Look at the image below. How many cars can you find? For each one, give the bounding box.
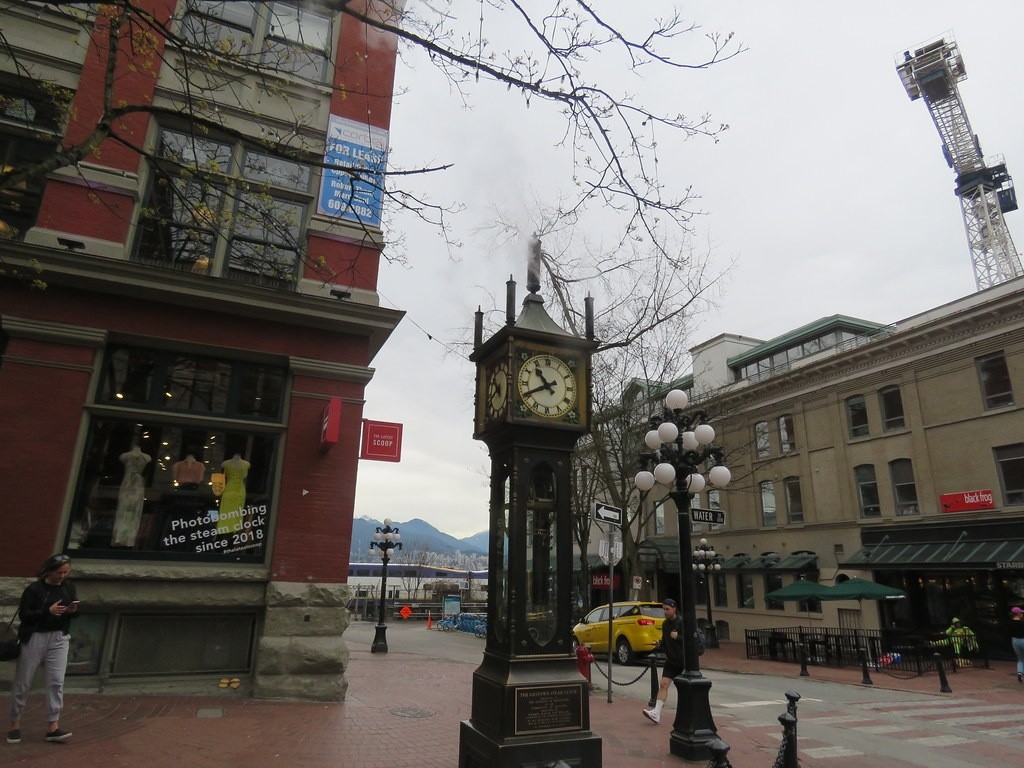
[571,601,681,665]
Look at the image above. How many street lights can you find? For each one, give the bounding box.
[632,388,732,760]
[367,519,402,656]
[691,536,722,649]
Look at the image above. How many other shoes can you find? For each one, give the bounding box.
[1017,672,1023,682]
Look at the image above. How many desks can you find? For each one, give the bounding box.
[796,638,824,663]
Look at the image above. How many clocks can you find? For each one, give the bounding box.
[513,340,586,427]
[485,351,508,427]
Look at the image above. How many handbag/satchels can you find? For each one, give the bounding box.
[0,621,20,662]
[671,616,705,656]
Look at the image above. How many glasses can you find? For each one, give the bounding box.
[49,554,70,562]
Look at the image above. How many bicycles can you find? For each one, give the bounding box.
[437,612,486,639]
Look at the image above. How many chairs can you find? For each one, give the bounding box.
[747,636,769,659]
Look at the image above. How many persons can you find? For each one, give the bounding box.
[172,455,205,483]
[930,618,978,658]
[7,553,80,743]
[110,447,152,547]
[217,454,250,534]
[1010,607,1024,682]
[642,598,682,724]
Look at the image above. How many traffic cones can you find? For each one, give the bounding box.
[427,610,431,629]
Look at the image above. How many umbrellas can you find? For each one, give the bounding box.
[765,578,906,628]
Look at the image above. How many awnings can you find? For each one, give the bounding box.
[839,539,1024,570]
[637,538,679,573]
[526,553,605,571]
[708,555,818,574]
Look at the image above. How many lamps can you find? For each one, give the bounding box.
[864,551,871,562]
[57,237,86,252]
[330,290,352,300]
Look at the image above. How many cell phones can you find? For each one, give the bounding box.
[67,601,80,608]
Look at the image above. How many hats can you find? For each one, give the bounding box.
[663,599,676,607]
[1012,607,1024,613]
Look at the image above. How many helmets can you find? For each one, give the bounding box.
[953,618,960,623]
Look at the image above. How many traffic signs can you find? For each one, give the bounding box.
[593,504,623,525]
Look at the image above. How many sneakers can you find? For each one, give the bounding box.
[45,728,72,741]
[642,709,660,723]
[6,729,22,744]
